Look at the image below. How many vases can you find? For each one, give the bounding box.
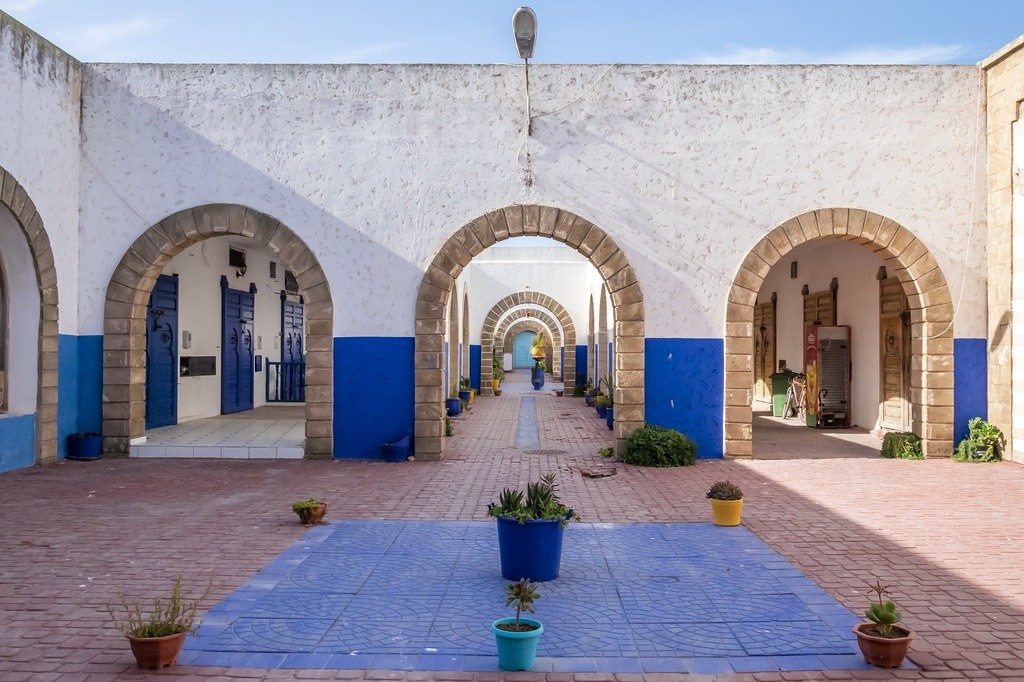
[493,390,502,396]
[382,434,411,463]
[65,429,104,460]
[556,390,564,396]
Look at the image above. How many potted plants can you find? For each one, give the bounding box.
[460,378,477,402]
[621,427,701,467]
[103,564,221,670]
[850,577,916,667]
[487,469,582,581]
[290,497,328,524]
[491,576,546,672]
[529,329,549,390]
[583,366,614,431]
[446,384,462,416]
[878,430,927,460]
[949,416,1005,464]
[705,478,747,527]
[493,354,505,390]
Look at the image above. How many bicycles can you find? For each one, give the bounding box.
[779,367,807,422]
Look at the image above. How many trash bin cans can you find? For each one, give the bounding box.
[65,432,103,459]
[769,371,800,418]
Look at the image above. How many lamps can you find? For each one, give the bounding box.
[236,261,248,279]
[511,5,544,137]
